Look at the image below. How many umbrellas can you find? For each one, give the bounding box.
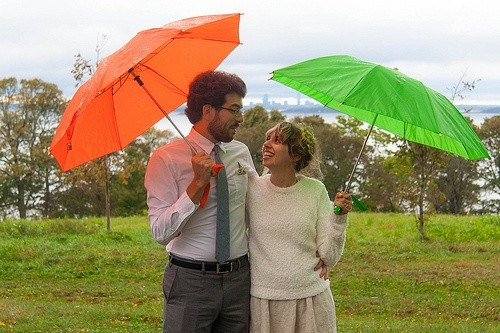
[48,12,244,210]
[267,55,491,215]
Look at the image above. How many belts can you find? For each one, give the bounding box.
[168,254,248,273]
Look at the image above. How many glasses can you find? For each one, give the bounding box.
[213,106,241,116]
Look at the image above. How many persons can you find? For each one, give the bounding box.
[245,122,352,333]
[143,70,338,333]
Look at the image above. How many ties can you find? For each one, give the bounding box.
[213,144,231,265]
